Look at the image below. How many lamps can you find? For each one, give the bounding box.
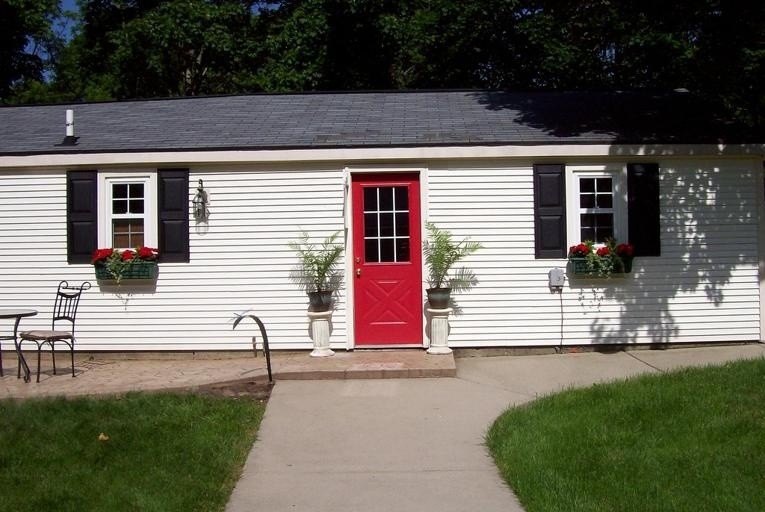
[190,179,210,222]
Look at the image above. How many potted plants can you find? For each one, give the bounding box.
[285,226,348,312]
[419,219,483,310]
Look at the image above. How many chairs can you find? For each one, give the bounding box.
[17,280,92,384]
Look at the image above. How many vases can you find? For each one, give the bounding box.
[95,259,157,282]
[569,256,632,277]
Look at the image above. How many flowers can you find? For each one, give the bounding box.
[90,247,158,287]
[568,239,633,282]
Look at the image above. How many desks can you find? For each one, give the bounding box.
[0,307,38,383]
